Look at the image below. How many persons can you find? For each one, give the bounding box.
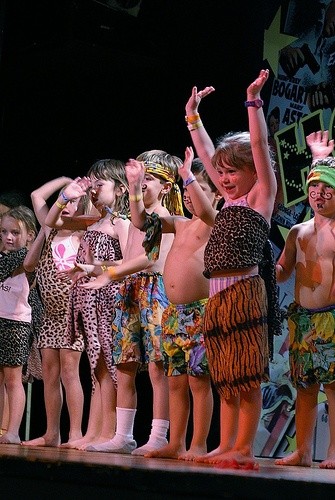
[20,175,86,448]
[275,157,335,470]
[0,205,46,444]
[45,159,131,451]
[267,106,283,153]
[57,150,182,456]
[124,147,223,461]
[185,69,277,465]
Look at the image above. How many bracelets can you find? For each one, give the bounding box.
[101,265,107,273]
[187,121,203,131]
[61,192,71,202]
[185,113,200,123]
[109,268,120,281]
[128,194,143,201]
[183,175,197,187]
[55,201,66,209]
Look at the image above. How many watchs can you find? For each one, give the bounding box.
[244,99,264,108]
